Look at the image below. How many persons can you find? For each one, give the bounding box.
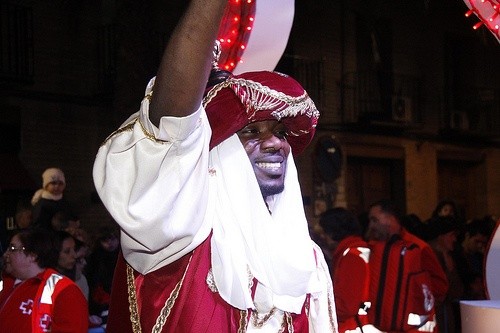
[402,202,500,290]
[0,206,37,249]
[66,215,89,247]
[0,226,88,333]
[92,226,118,328]
[32,168,71,252]
[319,206,372,333]
[368,201,449,333]
[47,232,105,332]
[91,0,337,333]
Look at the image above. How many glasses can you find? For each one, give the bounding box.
[7,244,26,253]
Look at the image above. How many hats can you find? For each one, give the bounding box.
[197,39,320,158]
[42,167,65,189]
